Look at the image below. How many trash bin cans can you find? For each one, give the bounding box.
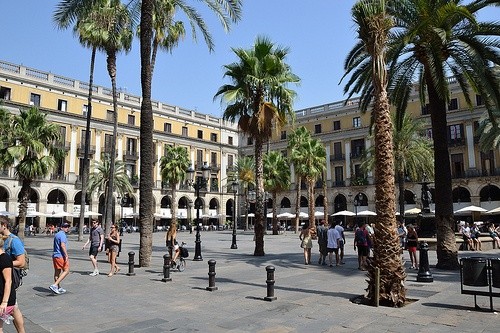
[461,256,489,287]
[491,257,500,289]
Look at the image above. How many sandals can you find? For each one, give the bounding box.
[114,267,121,275]
[108,273,113,277]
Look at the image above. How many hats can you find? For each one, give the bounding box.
[110,226,115,228]
[321,220,326,224]
[61,223,71,227]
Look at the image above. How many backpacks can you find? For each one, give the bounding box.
[9,235,30,278]
[4,253,23,290]
[355,229,366,244]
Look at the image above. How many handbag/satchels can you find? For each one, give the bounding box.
[300,242,304,248]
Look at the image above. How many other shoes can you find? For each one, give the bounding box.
[319,260,327,265]
[173,260,176,264]
[89,271,99,277]
[470,247,475,251]
[476,248,482,251]
[329,263,339,267]
[340,261,346,264]
[358,267,366,271]
[410,266,419,270]
[305,261,311,265]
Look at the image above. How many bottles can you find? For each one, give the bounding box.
[0,312,15,324]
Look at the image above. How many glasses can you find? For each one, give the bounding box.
[92,222,96,224]
[63,225,70,228]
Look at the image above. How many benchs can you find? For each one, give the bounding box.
[456,237,495,243]
[418,238,437,242]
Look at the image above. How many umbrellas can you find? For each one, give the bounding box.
[27,208,106,219]
[197,205,500,219]
[126,213,162,226]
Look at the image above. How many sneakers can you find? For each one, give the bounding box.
[49,284,67,295]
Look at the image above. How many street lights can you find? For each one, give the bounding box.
[230,178,239,249]
[347,191,363,230]
[185,161,213,262]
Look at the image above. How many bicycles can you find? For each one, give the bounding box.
[169,242,186,272]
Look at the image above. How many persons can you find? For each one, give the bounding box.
[48,222,71,295]
[166,222,180,265]
[0,234,25,333]
[0,214,25,268]
[106,222,121,277]
[461,221,500,251]
[81,218,104,276]
[28,223,88,236]
[298,219,421,271]
